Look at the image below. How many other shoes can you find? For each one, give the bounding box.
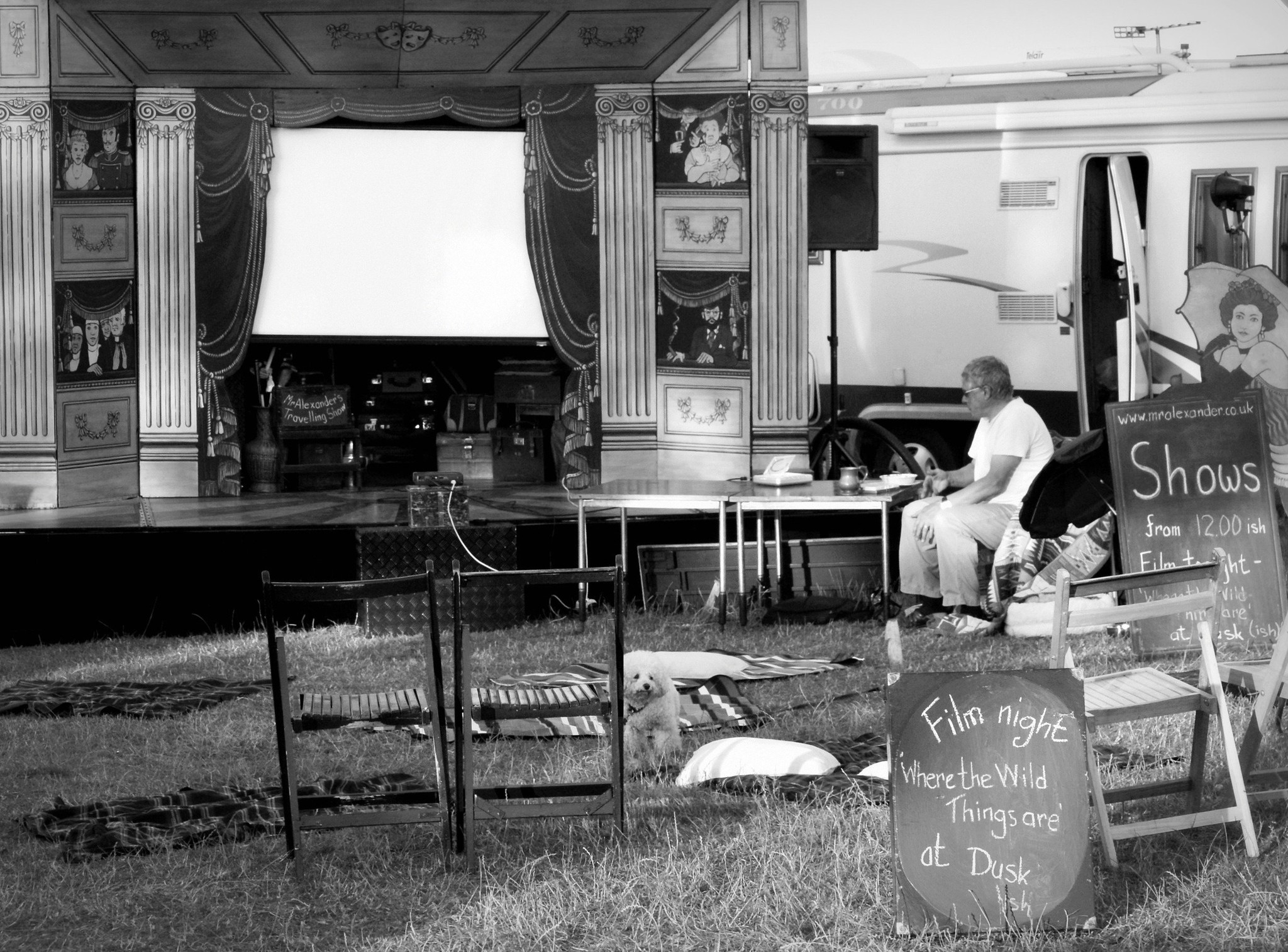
[885,607,932,628]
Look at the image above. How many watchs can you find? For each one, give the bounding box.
[940,497,951,510]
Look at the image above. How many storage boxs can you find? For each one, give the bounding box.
[273,384,351,431]
[436,360,561,481]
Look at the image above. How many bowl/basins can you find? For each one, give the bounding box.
[879,473,918,485]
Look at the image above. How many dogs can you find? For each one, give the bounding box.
[606,651,683,776]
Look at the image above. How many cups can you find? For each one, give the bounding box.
[904,392,912,406]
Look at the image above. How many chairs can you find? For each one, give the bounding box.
[1052,548,1262,869]
[452,555,628,874]
[258,558,452,870]
[1212,610,1288,831]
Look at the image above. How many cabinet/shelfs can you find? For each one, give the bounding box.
[278,422,364,493]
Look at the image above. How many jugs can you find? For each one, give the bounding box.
[838,465,869,496]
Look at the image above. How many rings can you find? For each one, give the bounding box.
[924,525,928,529]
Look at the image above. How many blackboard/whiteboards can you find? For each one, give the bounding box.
[1104,388,1286,656]
[880,665,1101,936]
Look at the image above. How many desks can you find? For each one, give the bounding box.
[570,478,924,633]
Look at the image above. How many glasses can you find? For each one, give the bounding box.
[963,387,982,398]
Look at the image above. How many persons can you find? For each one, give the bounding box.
[894,356,1051,631]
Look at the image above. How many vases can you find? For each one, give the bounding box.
[247,405,281,492]
